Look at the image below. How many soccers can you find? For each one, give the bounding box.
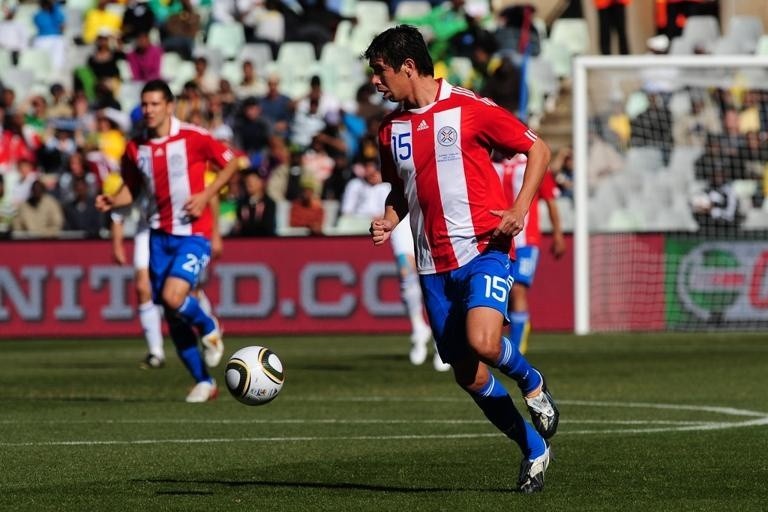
[225,346,284,405]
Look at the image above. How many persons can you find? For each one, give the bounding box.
[362,23,560,494]
[386,214,452,372]
[118,103,225,371]
[93,76,240,403]
[489,118,567,357]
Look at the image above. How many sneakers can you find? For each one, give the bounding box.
[184,377,217,404]
[139,353,166,370]
[197,289,211,316]
[432,345,451,372]
[411,328,430,366]
[200,316,224,368]
[521,366,559,438]
[517,437,553,492]
